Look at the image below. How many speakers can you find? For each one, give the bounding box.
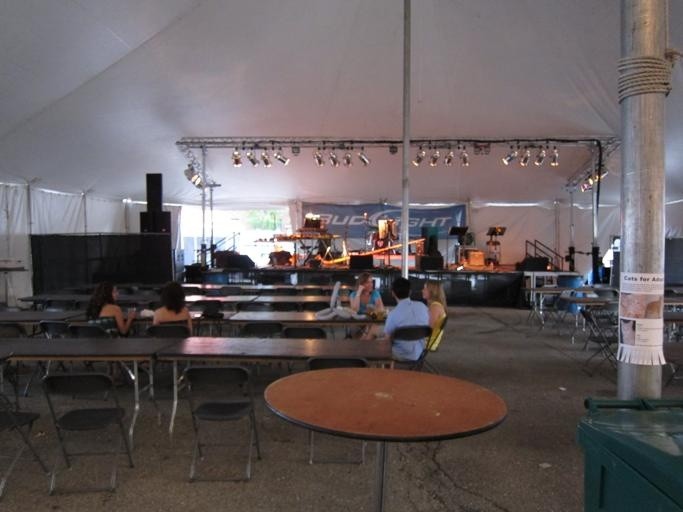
[139,211,154,233]
[146,173,163,212]
[155,211,171,233]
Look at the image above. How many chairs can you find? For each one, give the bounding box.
[191,300,221,310]
[270,302,301,332]
[279,327,327,373]
[578,308,618,377]
[182,286,198,294]
[217,286,243,296]
[420,314,450,374]
[273,287,298,295]
[40,369,134,493]
[159,325,192,425]
[302,300,329,312]
[304,356,369,369]
[301,287,323,295]
[0,359,52,499]
[374,324,433,369]
[182,366,262,481]
[238,322,282,336]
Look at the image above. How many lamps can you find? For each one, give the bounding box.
[550,148,559,166]
[445,151,455,166]
[580,167,608,193]
[185,168,197,182]
[312,151,325,168]
[411,150,426,167]
[520,150,530,167]
[356,152,370,166]
[260,152,272,167]
[460,151,471,168]
[194,176,201,187]
[273,152,290,166]
[231,151,242,168]
[328,151,339,168]
[246,153,259,167]
[342,153,351,166]
[501,150,517,167]
[430,151,440,168]
[533,150,547,166]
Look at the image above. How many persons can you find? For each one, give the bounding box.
[85,281,137,338]
[421,281,449,351]
[152,282,193,337]
[350,273,385,339]
[366,277,430,363]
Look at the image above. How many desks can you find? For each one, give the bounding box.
[260,284,350,295]
[121,308,236,322]
[0,337,175,464]
[185,294,255,306]
[155,335,394,447]
[0,261,29,310]
[554,297,611,330]
[228,309,385,324]
[67,283,99,292]
[526,288,589,326]
[17,292,95,310]
[180,283,260,294]
[254,294,332,305]
[264,366,508,511]
[2,305,87,338]
